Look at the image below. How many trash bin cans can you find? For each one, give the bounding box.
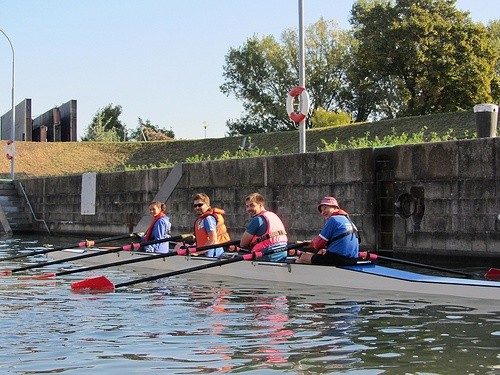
[473,103,499,137]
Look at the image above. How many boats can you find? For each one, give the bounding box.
[46,246,500,308]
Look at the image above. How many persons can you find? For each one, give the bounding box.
[133,201,172,254]
[68,273,360,375]
[239,192,288,262]
[174,193,231,258]
[295,196,360,266]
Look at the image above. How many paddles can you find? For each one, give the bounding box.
[69,240,308,295]
[16,239,236,281]
[358,251,500,282]
[0,233,193,277]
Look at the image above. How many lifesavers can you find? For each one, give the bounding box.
[5,140,15,160]
[285,86,310,123]
